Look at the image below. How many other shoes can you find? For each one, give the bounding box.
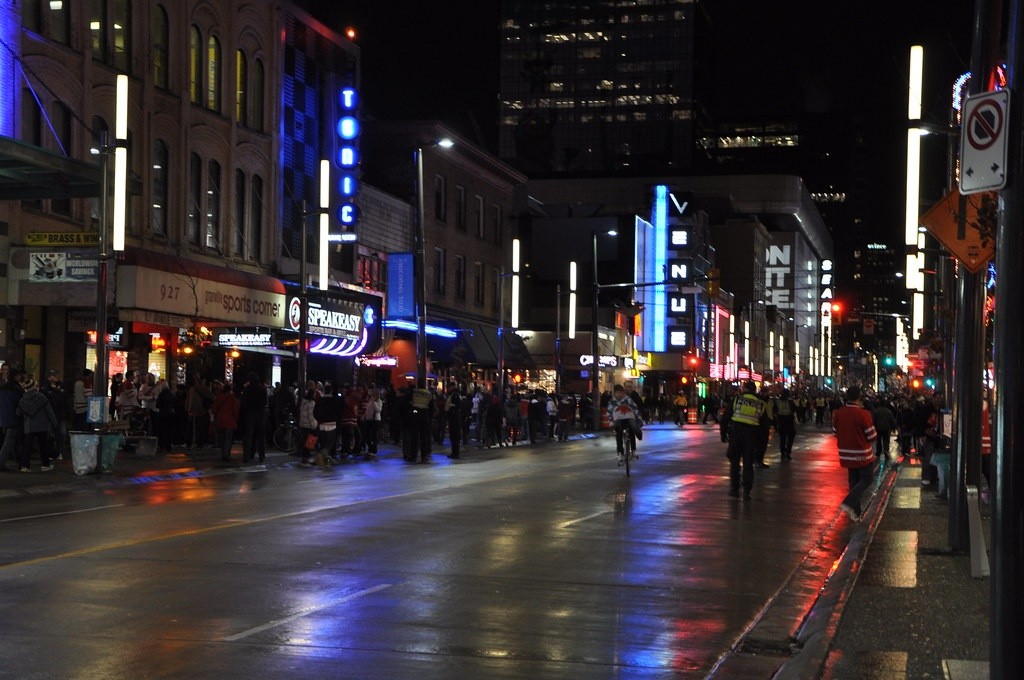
[780,447,790,456]
[21,466,32,473]
[840,503,859,522]
[632,450,635,456]
[289,443,379,468]
[730,487,739,497]
[433,436,570,458]
[755,463,769,469]
[743,488,750,497]
[41,465,55,472]
[618,452,625,462]
[637,433,642,440]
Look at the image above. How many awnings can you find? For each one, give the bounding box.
[451,318,537,368]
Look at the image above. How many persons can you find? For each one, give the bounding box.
[608,384,642,467]
[597,383,947,469]
[834,386,876,522]
[0,361,266,473]
[721,382,766,503]
[270,379,594,468]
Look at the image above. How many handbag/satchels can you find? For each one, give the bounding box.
[303,433,318,450]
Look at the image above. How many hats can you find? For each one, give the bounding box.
[134,371,142,377]
[25,379,38,392]
[48,369,56,376]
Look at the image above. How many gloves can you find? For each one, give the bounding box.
[609,422,614,428]
[720,433,728,442]
[638,416,642,427]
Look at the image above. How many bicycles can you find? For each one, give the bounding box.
[613,423,639,478]
[272,407,302,453]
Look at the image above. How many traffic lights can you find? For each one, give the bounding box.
[690,358,696,364]
[831,304,841,311]
[925,379,934,387]
[912,380,922,388]
[886,358,892,365]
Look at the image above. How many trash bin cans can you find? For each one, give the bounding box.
[934,448,950,498]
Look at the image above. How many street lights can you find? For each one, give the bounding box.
[590,228,618,432]
[413,139,455,462]
[87,145,110,478]
[894,44,972,554]
[556,261,578,392]
[496,239,519,446]
